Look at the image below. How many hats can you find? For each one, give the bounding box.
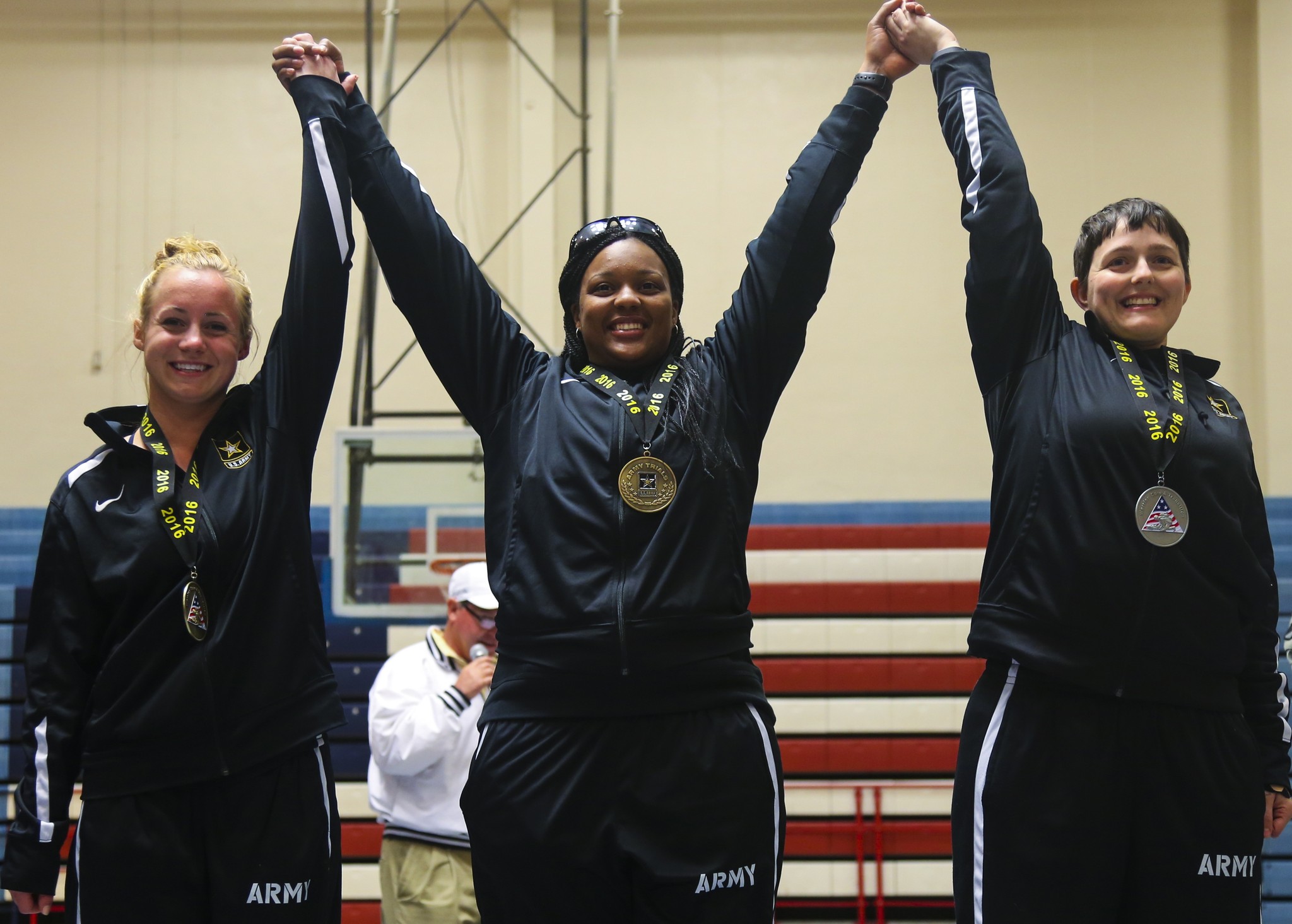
[449,561,499,610]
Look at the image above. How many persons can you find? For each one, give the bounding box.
[367,561,499,924]
[0,32,358,924]
[274,0,928,924]
[885,0,1292,924]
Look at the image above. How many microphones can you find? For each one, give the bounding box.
[470,643,488,660]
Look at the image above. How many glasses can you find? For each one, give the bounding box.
[463,601,497,630]
[569,216,669,258]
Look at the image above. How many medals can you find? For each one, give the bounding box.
[618,456,677,512]
[183,581,209,641]
[1135,486,1189,547]
